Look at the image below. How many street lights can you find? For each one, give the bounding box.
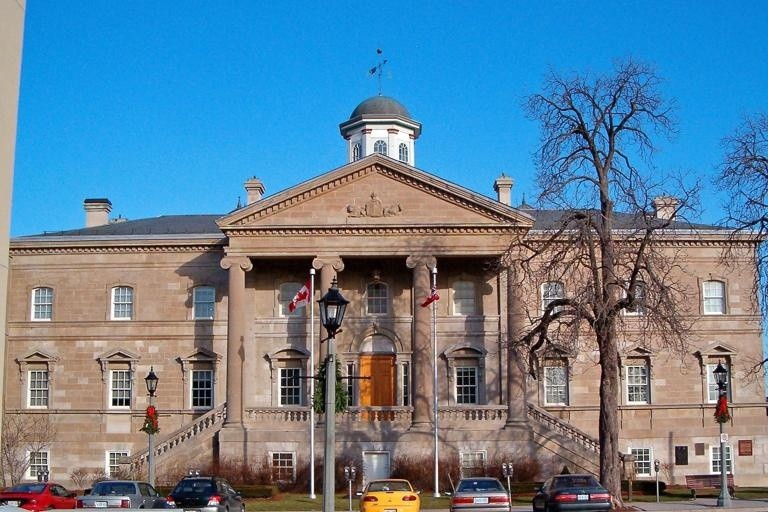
[712,358,733,507]
[144,365,159,488]
[315,273,351,511]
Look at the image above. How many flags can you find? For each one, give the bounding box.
[419,281,441,307]
[288,276,316,314]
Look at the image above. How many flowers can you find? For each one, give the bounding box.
[139,405,160,434]
[713,395,730,423]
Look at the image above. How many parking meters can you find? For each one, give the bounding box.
[344,466,356,511]
[502,462,514,511]
[37,469,50,483]
[654,460,660,500]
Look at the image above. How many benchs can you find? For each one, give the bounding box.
[686,475,735,500]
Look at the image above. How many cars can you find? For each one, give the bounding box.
[74,480,167,509]
[355,479,421,512]
[1,482,84,512]
[532,473,612,511]
[165,476,246,512]
[444,475,510,512]
